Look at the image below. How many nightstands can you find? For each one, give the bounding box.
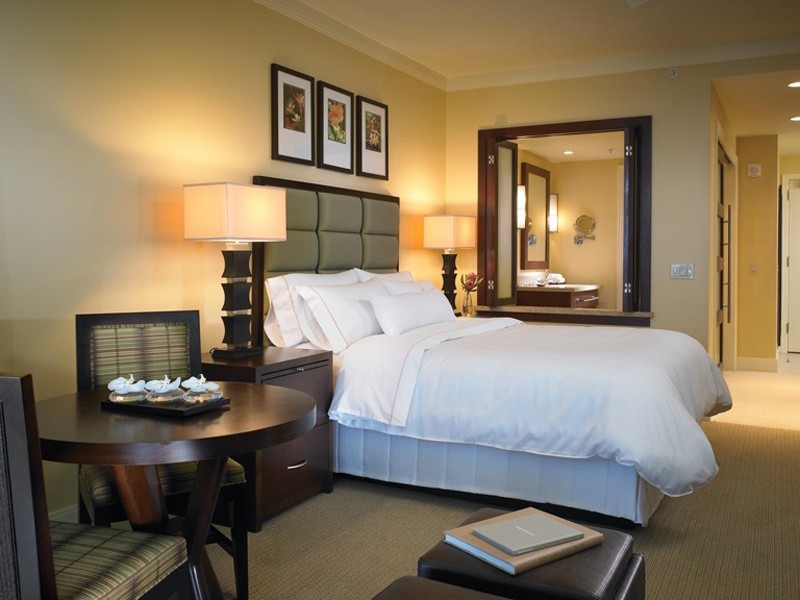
[198,346,334,532]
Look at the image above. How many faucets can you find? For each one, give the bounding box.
[536,278,559,286]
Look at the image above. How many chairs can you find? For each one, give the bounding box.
[0,373,188,600]
[75,310,248,600]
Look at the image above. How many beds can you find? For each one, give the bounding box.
[251,176,733,535]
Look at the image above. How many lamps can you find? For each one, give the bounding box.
[183,182,287,360]
[423,216,478,310]
[573,214,596,244]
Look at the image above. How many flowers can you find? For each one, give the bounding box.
[459,271,484,314]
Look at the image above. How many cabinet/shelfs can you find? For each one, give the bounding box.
[515,284,600,308]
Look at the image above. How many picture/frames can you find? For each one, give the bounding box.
[355,95,389,180]
[317,80,354,174]
[269,63,316,166]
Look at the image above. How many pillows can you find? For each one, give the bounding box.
[264,266,457,354]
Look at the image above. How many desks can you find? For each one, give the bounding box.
[31,381,317,600]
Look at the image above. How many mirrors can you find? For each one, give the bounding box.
[521,162,550,270]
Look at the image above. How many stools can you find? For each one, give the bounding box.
[371,507,646,600]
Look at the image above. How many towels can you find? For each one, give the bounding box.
[547,273,566,284]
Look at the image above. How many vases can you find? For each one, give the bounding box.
[462,293,475,317]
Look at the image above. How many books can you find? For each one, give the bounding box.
[442,506,604,576]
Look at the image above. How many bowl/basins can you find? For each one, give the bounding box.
[183,388,223,403]
[109,388,148,402]
[146,387,185,403]
